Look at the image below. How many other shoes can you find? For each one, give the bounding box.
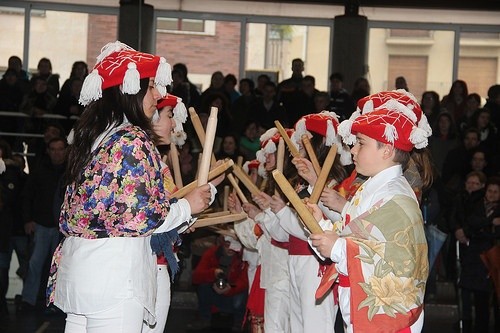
[18,303,33,313]
[187,319,210,329]
[232,317,241,332]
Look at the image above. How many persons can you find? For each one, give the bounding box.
[46,40,212,333]
[0,56,89,333]
[142,58,371,333]
[297,99,429,333]
[395,75,500,333]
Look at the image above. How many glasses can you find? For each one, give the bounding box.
[423,97,433,101]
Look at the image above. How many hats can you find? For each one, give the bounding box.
[78,41,174,107]
[242,160,260,175]
[291,110,353,166]
[149,93,188,132]
[256,128,294,162]
[336,89,433,151]
